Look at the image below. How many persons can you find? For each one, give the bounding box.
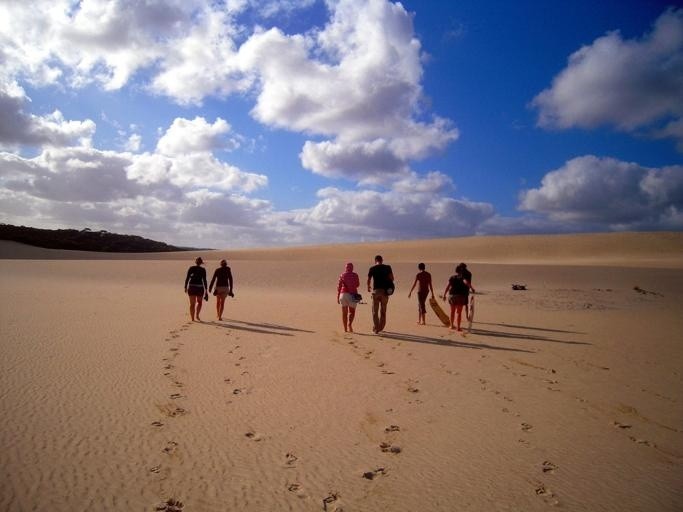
[408,263,433,325]
[337,263,360,331]
[185,257,208,321]
[443,266,475,331]
[209,260,234,320]
[367,255,394,334]
[460,263,471,320]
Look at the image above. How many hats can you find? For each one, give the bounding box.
[195,257,204,264]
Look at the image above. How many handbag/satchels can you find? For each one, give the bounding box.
[340,275,362,303]
